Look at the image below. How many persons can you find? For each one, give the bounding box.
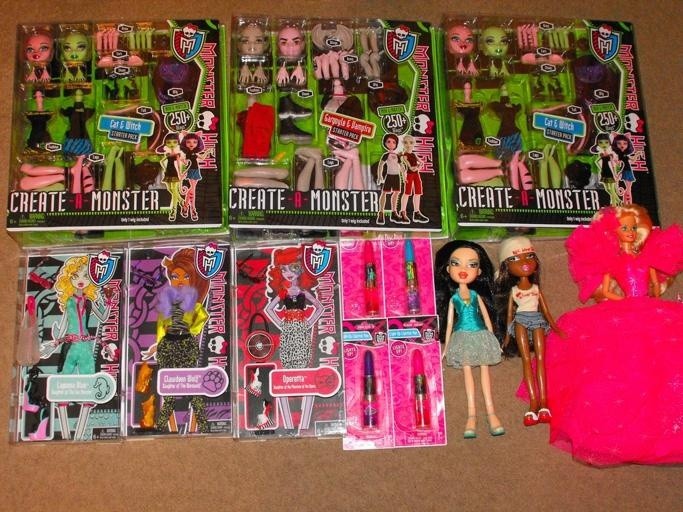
[492,236,569,425]
[232,27,383,190]
[434,242,512,438]
[515,203,683,467]
[448,24,572,190]
[19,25,154,191]
[9,16,683,472]
